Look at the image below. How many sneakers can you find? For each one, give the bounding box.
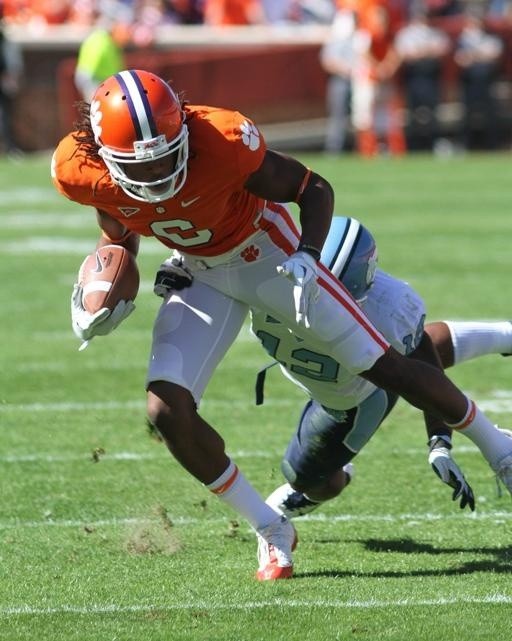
[263,464,356,519]
[486,425,512,499]
[255,519,299,582]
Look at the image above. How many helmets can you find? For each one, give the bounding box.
[319,215,377,302]
[90,70,188,207]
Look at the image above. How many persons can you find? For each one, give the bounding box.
[260,214,511,522]
[1,0,511,154]
[49,68,511,588]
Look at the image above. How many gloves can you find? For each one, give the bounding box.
[71,284,136,341]
[428,451,477,513]
[153,257,191,296]
[277,253,321,330]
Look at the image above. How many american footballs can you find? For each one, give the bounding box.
[77,245,138,314]
